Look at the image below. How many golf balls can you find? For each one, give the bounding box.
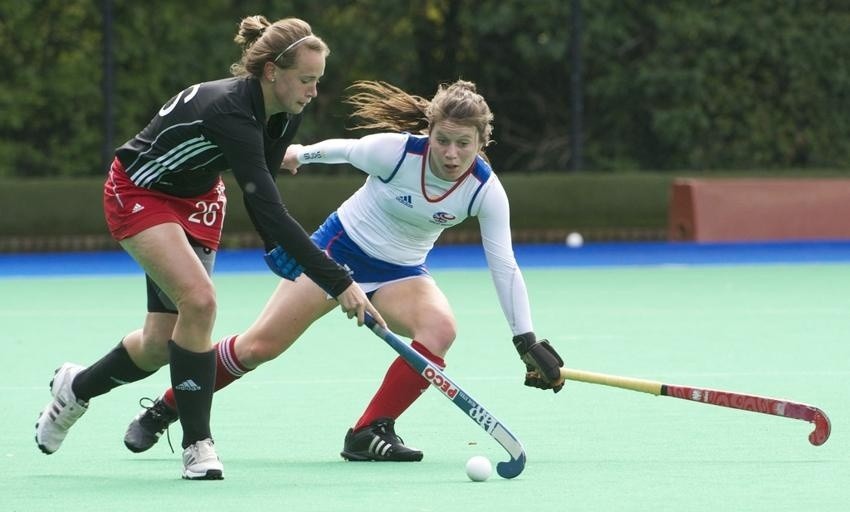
[566,233,583,249]
[464,456,491,482]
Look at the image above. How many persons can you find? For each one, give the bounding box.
[33,14,387,480]
[124,81,565,462]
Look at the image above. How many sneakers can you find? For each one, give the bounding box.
[123,396,179,454]
[33,362,89,456]
[340,418,423,462]
[180,437,224,480]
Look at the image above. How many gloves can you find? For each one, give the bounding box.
[264,245,306,282]
[512,331,566,394]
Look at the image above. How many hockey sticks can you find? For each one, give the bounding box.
[354,310,526,478]
[528,365,831,446]
